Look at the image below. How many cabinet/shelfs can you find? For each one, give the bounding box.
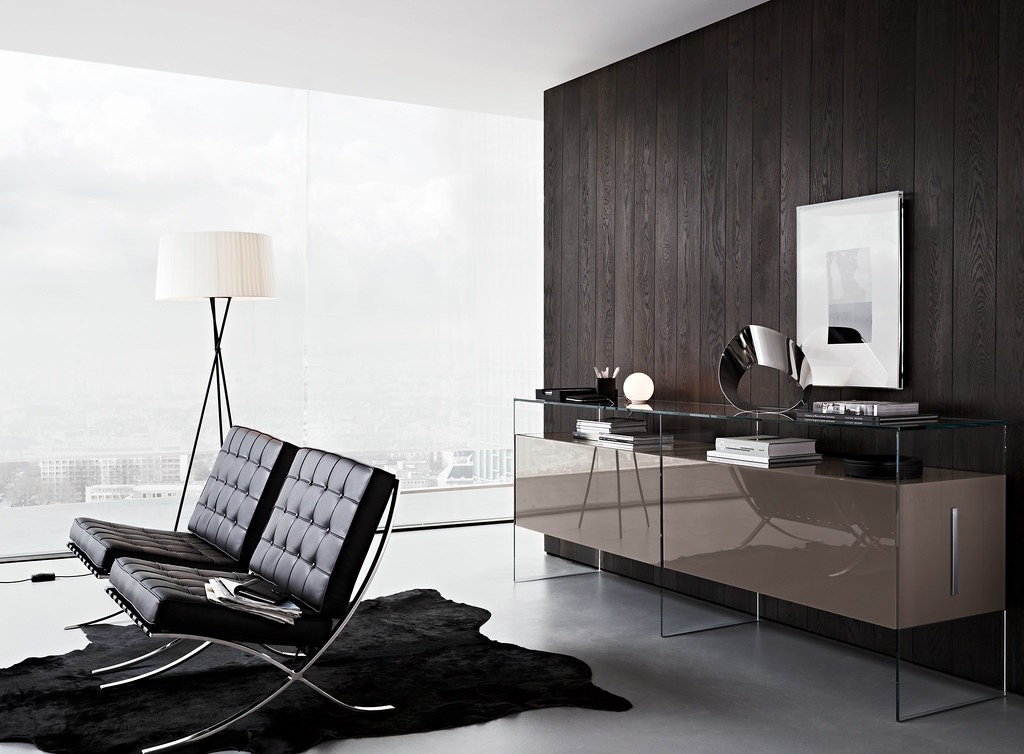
[513,397,1008,726]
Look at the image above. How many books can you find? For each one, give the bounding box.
[203,576,303,626]
[565,395,609,404]
[572,418,674,452]
[796,399,938,424]
[707,434,823,470]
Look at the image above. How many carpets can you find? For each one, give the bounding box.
[1,588,636,753]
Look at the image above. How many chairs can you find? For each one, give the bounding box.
[99,447,397,754]
[67,425,301,676]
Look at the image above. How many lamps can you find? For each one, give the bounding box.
[152,229,276,534]
